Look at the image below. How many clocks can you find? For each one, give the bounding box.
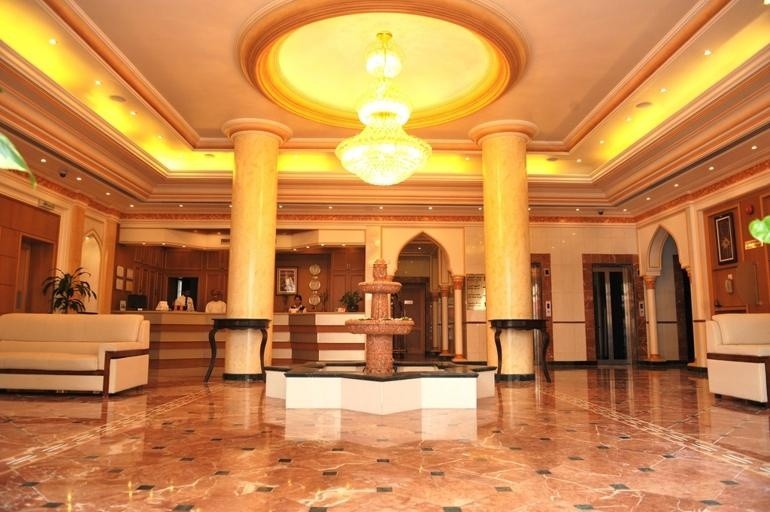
[308,264,322,306]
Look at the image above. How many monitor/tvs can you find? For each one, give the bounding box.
[128,294,147,310]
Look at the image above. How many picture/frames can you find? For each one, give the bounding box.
[276,266,299,296]
[714,211,737,266]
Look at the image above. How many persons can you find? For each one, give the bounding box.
[174,287,194,311]
[205,288,227,313]
[288,294,308,313]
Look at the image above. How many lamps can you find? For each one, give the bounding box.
[333,32,433,187]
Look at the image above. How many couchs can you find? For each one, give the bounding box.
[704,312,769,407]
[0,313,150,396]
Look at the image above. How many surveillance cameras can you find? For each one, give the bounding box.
[597,209,604,215]
[59,170,68,177]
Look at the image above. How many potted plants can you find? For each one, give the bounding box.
[339,289,362,311]
